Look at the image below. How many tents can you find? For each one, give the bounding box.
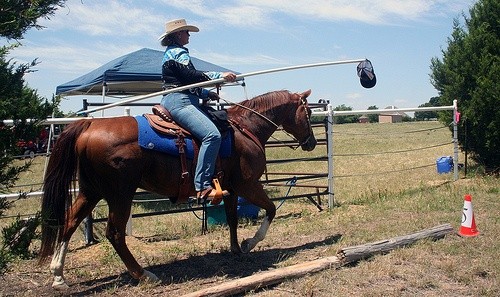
[40,47,250,193]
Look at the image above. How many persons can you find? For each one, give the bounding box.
[159,18,237,204]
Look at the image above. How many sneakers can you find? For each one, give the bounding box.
[197,187,230,204]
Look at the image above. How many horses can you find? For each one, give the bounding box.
[35,89,317,290]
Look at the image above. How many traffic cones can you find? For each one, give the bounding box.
[459,194,481,237]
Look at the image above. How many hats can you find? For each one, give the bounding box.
[159,19,199,40]
[357,59,376,88]
